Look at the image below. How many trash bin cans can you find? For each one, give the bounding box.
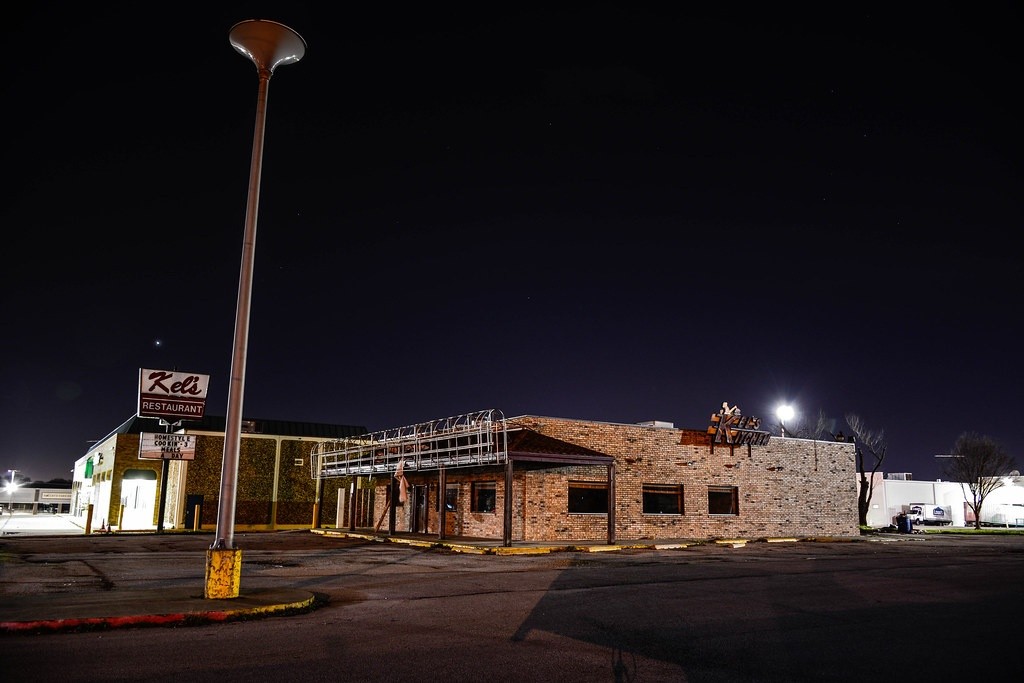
[897,517,910,534]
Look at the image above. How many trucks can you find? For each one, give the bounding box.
[905,503,953,525]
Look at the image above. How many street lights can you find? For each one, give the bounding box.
[206,18,307,599]
[7,469,21,515]
[776,406,794,437]
